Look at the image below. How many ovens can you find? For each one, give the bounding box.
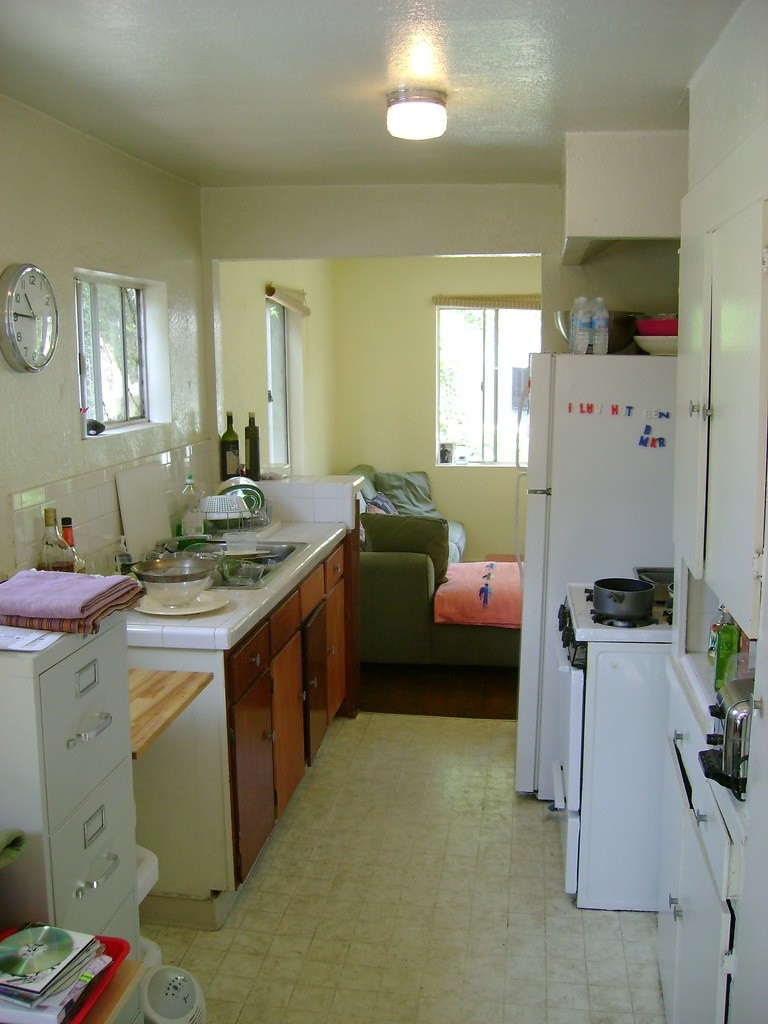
[551,642,586,893]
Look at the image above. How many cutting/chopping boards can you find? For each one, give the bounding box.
[127,668,215,760]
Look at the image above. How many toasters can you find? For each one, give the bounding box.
[698,676,754,802]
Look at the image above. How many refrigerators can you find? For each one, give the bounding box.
[512,353,678,804]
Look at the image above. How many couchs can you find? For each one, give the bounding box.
[343,464,520,667]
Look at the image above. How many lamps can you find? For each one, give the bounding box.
[386,86,448,140]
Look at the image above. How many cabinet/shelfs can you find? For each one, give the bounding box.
[0,607,141,962]
[666,121,768,1024]
[127,539,347,932]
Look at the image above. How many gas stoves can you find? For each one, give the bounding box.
[557,582,672,668]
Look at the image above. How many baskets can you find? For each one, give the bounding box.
[203,495,253,520]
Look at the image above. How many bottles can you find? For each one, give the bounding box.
[220,411,240,481]
[174,474,208,537]
[569,296,609,355]
[714,608,739,693]
[244,412,260,481]
[707,605,726,667]
[61,516,86,575]
[36,508,75,573]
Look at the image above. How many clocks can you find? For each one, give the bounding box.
[0,263,59,373]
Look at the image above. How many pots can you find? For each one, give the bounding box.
[592,577,656,620]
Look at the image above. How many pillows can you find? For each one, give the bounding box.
[360,513,449,584]
[371,471,437,516]
[369,491,398,516]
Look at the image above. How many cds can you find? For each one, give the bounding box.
[0,926,74,975]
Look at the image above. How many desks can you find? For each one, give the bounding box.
[128,668,215,760]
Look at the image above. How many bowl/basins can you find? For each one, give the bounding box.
[222,558,265,586]
[635,336,678,356]
[554,310,646,354]
[223,531,257,554]
[182,543,224,569]
[635,320,678,335]
[130,556,219,609]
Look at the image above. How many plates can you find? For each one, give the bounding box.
[217,484,265,519]
[133,590,230,616]
